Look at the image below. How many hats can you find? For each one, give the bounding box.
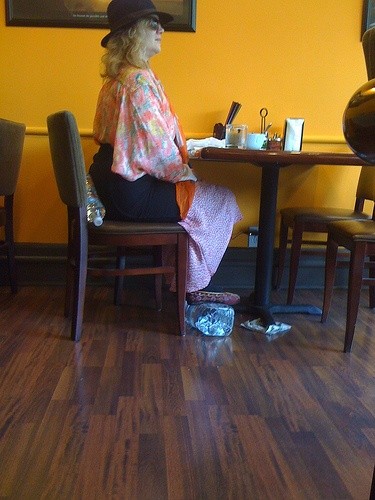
[101,0,174,48]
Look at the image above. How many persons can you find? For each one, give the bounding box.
[88,0,243,295]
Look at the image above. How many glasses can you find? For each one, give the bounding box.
[147,19,160,28]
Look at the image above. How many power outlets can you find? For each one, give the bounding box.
[248,226,258,248]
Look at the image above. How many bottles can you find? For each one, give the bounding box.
[184,303,234,337]
[85,174,106,226]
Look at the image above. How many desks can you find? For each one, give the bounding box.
[185,139,375,328]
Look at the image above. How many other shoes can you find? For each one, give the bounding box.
[186,290,241,305]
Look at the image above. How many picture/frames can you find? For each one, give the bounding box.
[5,0,196,32]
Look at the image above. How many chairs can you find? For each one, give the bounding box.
[47,110,189,342]
[274,165,375,305]
[0,118,26,295]
[321,207,375,353]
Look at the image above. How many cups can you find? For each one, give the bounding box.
[248,133,268,150]
[225,124,247,150]
